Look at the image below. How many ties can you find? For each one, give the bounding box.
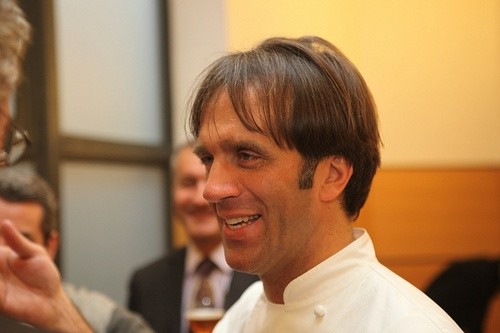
[194,258,215,309]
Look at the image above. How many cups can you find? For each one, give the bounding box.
[186,308,223,333]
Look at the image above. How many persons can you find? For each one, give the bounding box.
[0,35,469,333]
[0,160,155,333]
[127,141,261,333]
[0,0,34,169]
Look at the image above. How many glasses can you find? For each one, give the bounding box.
[0,105,34,166]
